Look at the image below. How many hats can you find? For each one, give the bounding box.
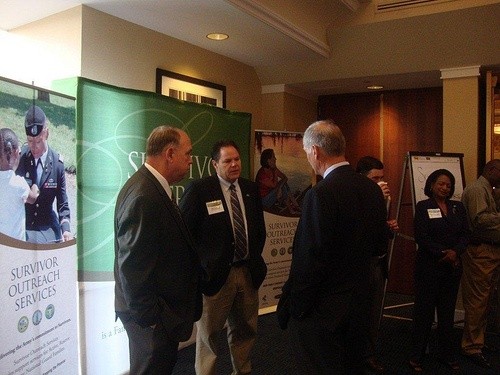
[25,105,46,137]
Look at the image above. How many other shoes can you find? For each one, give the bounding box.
[365,357,384,373]
[471,354,490,368]
[444,358,460,370]
[407,358,423,372]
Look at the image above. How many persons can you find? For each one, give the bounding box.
[0,104,73,244]
[178,140,266,375]
[409,169,469,373]
[114,125,204,375]
[255,149,291,210]
[275,120,399,375]
[461,160,500,371]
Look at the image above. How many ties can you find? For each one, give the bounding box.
[228,185,247,260]
[171,188,184,225]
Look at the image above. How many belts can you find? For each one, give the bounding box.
[481,239,499,246]
[231,260,248,266]
